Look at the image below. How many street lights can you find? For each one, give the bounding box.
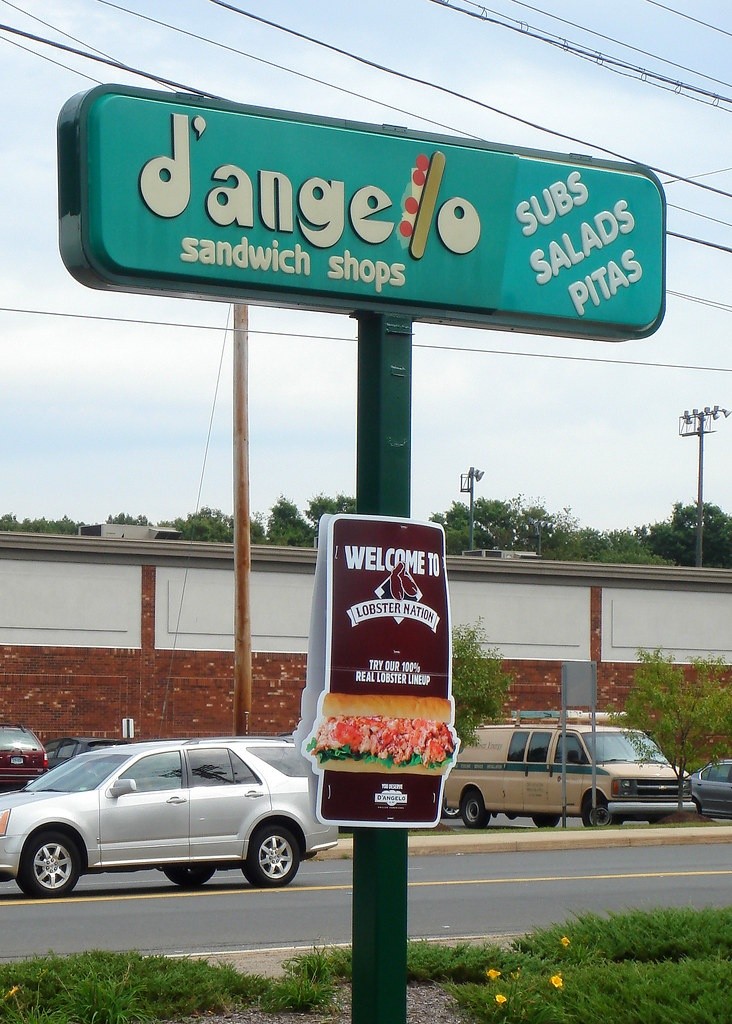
[680,405,732,566]
[461,467,486,550]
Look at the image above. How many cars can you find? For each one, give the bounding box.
[690,757,732,820]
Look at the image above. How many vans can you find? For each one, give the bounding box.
[443,722,699,832]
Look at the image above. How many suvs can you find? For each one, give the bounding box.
[42,734,131,778]
[0,734,340,900]
[0,722,50,790]
